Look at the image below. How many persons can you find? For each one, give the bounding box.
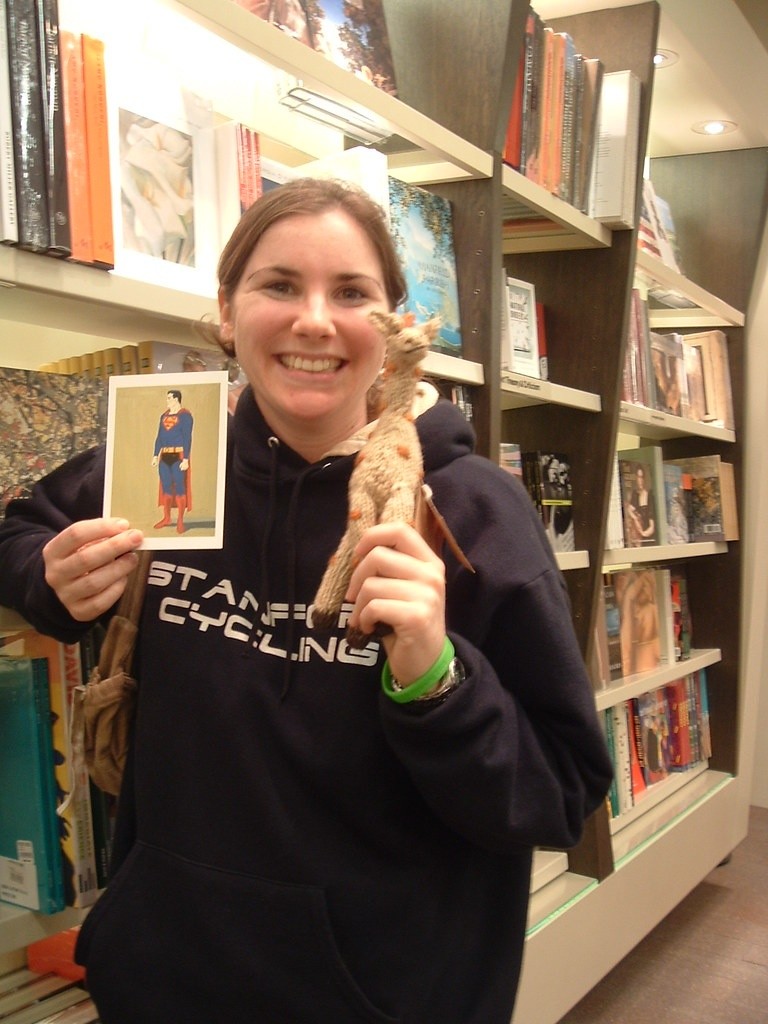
[0,177,613,1024]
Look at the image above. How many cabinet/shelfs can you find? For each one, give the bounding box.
[0,0,768,1024]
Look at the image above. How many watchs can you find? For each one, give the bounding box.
[391,657,465,701]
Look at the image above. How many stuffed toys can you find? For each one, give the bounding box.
[315,311,445,615]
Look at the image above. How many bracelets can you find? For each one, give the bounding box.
[382,634,455,703]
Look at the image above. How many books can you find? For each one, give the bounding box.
[594,566,695,693]
[0,0,465,358]
[0,608,115,921]
[436,267,736,430]
[498,442,739,551]
[0,339,247,497]
[600,668,712,817]
[499,0,687,277]
